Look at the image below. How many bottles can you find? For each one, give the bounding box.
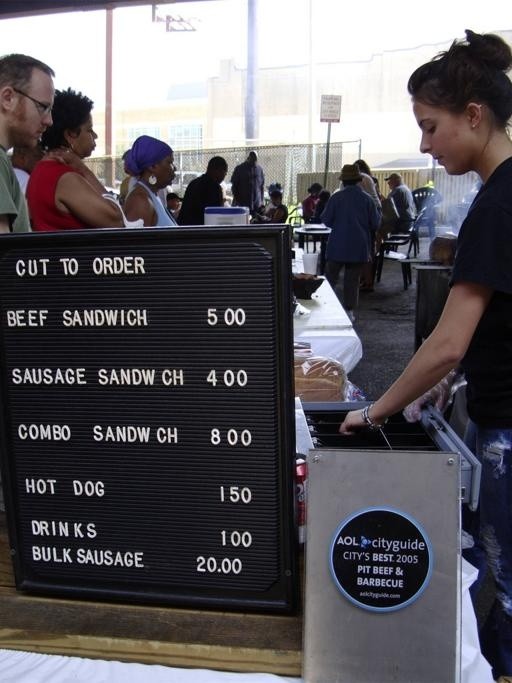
[297,201,303,216]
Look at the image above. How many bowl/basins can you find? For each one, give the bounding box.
[291,273,325,300]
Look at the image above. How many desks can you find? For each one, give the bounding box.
[294,224,340,267]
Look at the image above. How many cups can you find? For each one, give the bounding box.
[303,252,319,275]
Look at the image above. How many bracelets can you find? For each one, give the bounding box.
[363,402,388,431]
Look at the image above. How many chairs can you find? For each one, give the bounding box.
[409,186,444,236]
[373,211,420,289]
[389,205,429,260]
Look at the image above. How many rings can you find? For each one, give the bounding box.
[65,147,69,152]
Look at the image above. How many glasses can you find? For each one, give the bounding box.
[12,86,54,117]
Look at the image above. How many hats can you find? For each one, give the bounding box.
[338,165,364,181]
[384,173,400,180]
[268,183,283,195]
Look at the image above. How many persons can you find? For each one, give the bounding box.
[12,137,47,194]
[25,86,125,233]
[229,148,418,324]
[0,54,58,235]
[119,134,228,226]
[339,30,512,672]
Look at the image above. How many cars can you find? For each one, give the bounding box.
[93,170,271,207]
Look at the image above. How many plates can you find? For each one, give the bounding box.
[304,223,327,229]
